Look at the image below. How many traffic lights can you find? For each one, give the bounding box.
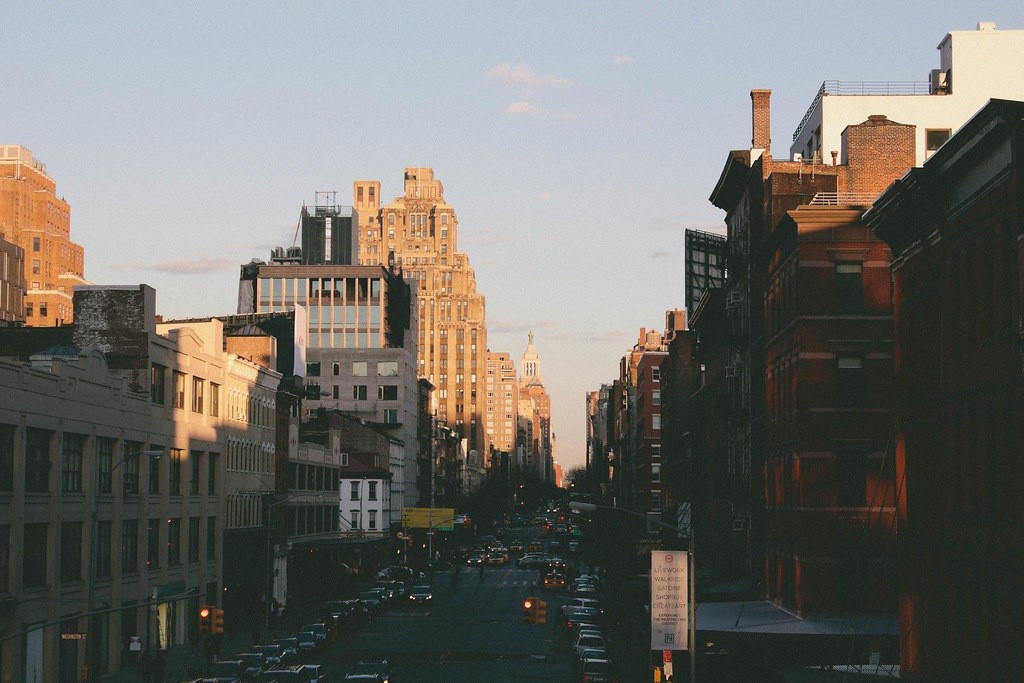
[197,605,211,633]
[520,597,549,626]
[211,606,224,635]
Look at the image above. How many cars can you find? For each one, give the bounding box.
[189,577,432,683]
[460,494,616,683]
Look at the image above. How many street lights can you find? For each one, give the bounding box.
[568,501,695,683]
[264,491,324,641]
[77,448,168,682]
